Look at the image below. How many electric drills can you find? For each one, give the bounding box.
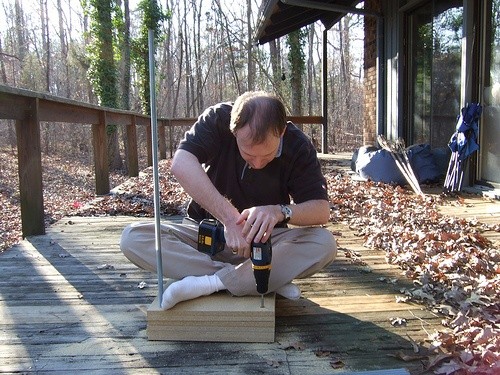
[197,219,272,307]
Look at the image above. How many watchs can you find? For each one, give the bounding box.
[278,204,292,224]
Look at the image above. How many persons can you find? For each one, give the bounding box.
[119,90,338,313]
